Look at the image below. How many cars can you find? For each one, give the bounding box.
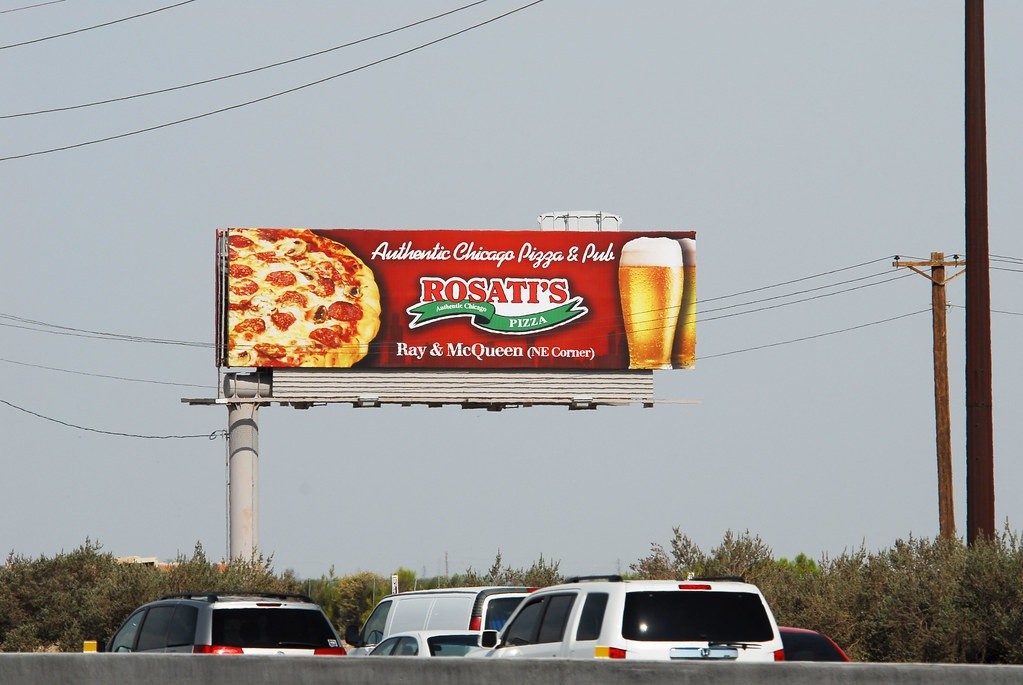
[779,626,851,662]
[367,629,486,658]
[346,586,547,656]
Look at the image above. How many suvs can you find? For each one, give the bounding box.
[478,574,787,663]
[106,591,347,655]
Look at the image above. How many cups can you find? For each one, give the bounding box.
[618,247,696,371]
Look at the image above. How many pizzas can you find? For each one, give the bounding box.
[228,227,381,368]
